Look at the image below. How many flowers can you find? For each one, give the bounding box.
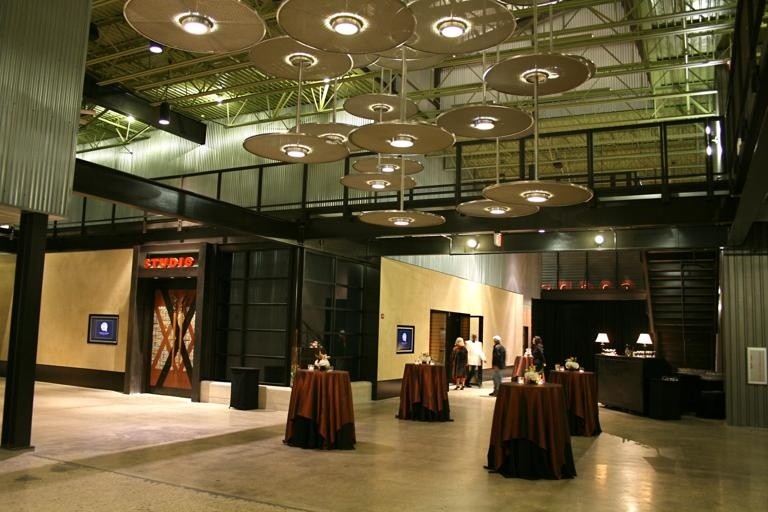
[413,352,432,365]
[522,365,547,385]
[308,340,336,371]
[564,356,580,370]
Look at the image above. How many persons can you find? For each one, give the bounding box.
[488,336,507,398]
[530,336,547,372]
[464,336,488,389]
[451,337,469,390]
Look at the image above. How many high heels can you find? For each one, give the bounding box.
[461,385,466,390]
[455,386,460,391]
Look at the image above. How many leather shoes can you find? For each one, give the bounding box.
[466,382,473,389]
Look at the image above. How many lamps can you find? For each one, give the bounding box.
[276,0,417,55]
[287,78,363,152]
[158,68,171,126]
[352,157,424,175]
[149,41,164,54]
[595,333,609,354]
[123,0,267,54]
[340,67,419,194]
[632,333,655,358]
[242,36,353,164]
[457,44,538,218]
[394,1,517,56]
[544,3,597,81]
[342,94,419,122]
[347,46,456,230]
[436,48,534,142]
[483,1,595,208]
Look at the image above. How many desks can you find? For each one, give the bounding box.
[483,382,577,479]
[550,370,603,437]
[395,362,455,422]
[511,355,534,382]
[281,368,357,450]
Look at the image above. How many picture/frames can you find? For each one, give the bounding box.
[86,314,120,345]
[746,346,768,384]
[396,325,416,354]
